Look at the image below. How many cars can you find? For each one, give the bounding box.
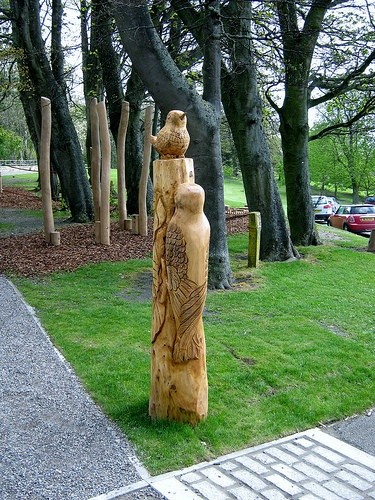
[311,195,375,233]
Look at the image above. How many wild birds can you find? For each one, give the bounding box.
[151,110,191,157]
